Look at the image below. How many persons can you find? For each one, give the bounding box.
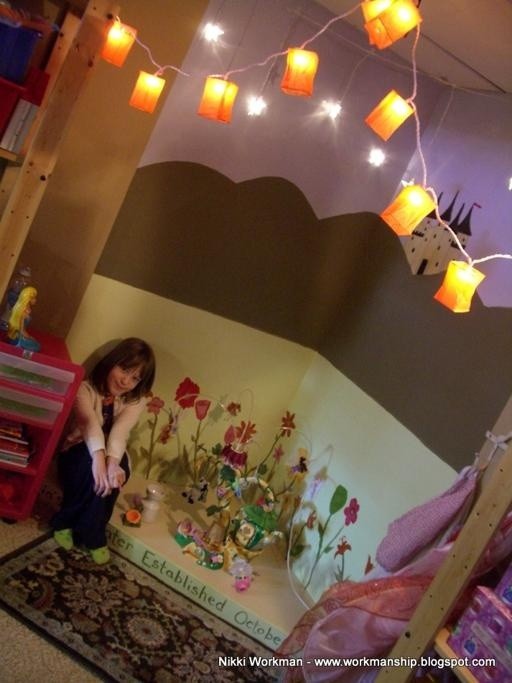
[5,285,44,352]
[50,336,157,567]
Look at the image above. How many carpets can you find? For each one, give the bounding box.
[1,527,287,682]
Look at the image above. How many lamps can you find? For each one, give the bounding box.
[99,1,512,318]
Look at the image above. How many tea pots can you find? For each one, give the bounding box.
[229,500,283,552]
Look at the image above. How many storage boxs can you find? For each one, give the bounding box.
[450,584,511,682]
[0,77,23,143]
[1,13,44,86]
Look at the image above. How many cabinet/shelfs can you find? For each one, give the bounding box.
[0,0,106,311]
[0,321,82,520]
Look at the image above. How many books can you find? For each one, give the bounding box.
[0,417,38,469]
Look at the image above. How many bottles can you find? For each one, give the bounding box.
[0,270,31,331]
[141,483,165,523]
